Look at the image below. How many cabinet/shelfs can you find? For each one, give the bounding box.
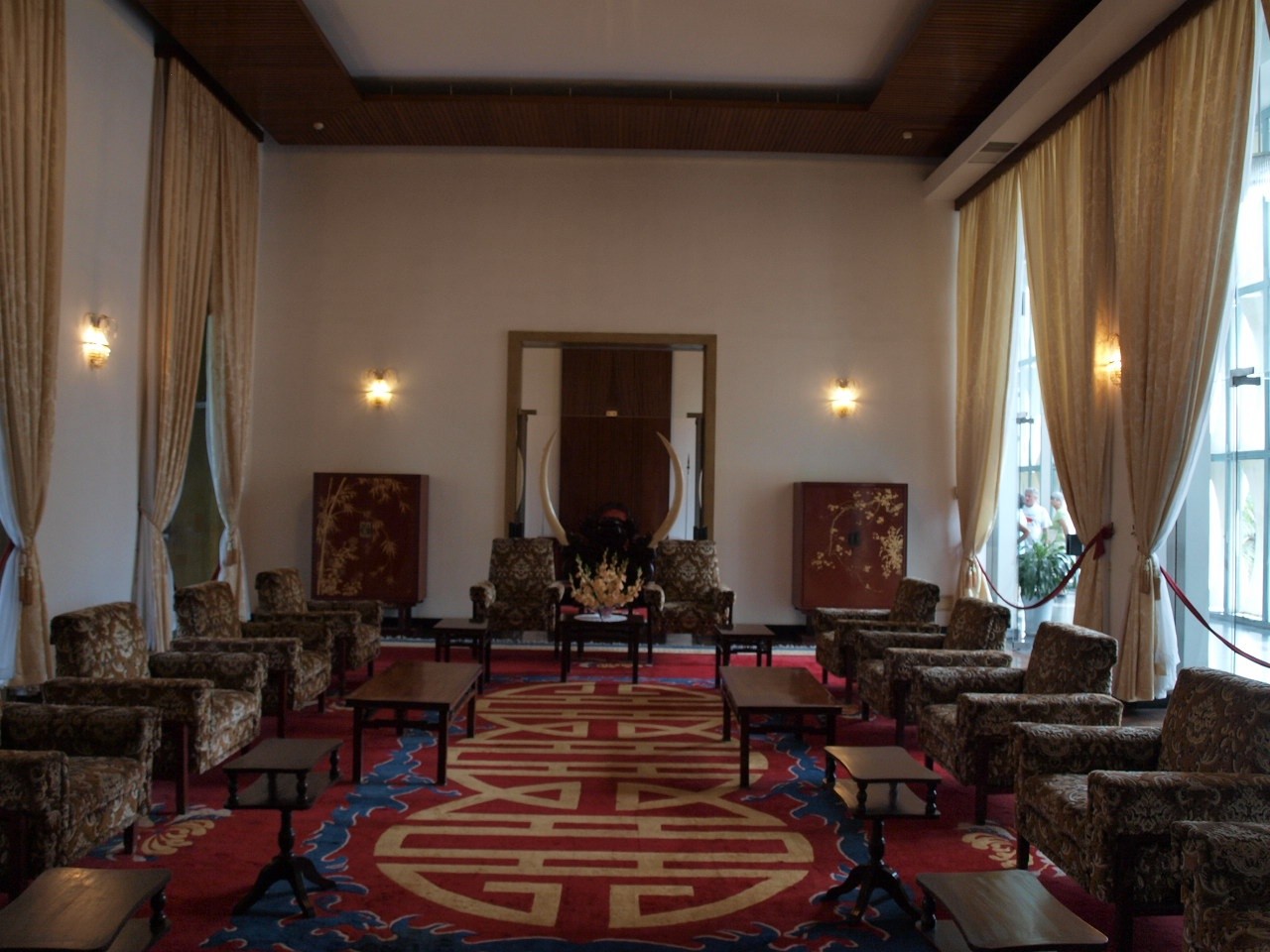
[312,473,428,624]
[793,482,908,623]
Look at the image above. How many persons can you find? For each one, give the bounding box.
[1024,487,1053,549]
[1019,493,1027,555]
[1050,492,1077,543]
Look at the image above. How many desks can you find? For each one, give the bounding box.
[719,666,842,789]
[345,660,482,785]
[715,623,775,687]
[223,737,343,920]
[0,867,174,952]
[434,619,489,694]
[915,869,1110,952]
[554,614,644,684]
[819,745,943,919]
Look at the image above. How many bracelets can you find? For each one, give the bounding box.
[1043,542,1049,547]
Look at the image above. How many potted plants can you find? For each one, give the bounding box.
[1018,543,1077,635]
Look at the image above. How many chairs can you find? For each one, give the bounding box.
[644,540,734,643]
[470,538,565,641]
[0,568,385,902]
[816,580,1270,952]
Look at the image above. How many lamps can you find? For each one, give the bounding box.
[830,378,860,418]
[81,312,118,371]
[365,367,396,408]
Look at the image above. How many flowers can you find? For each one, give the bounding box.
[569,548,644,619]
